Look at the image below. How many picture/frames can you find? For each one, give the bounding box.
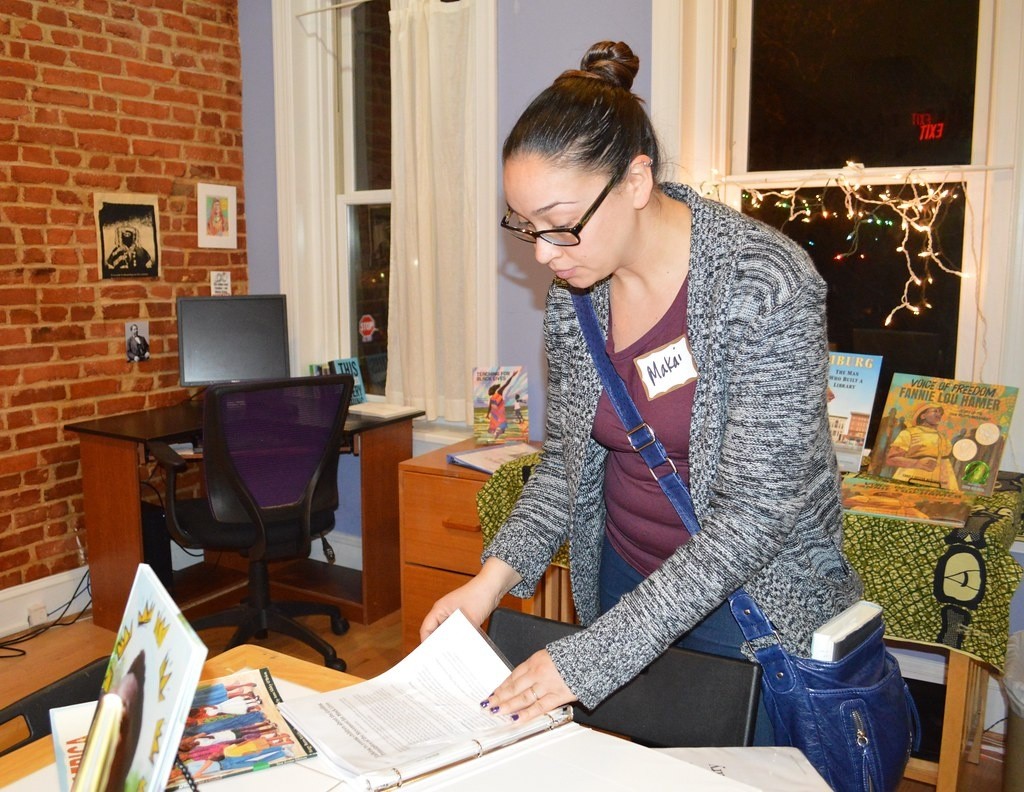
[196,182,238,250]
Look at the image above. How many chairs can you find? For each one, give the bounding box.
[487,607,762,748]
[155,372,355,671]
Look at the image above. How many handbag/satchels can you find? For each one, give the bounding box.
[762,620,921,792]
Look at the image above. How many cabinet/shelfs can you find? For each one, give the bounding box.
[396,438,544,659]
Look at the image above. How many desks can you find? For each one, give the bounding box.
[0,645,368,792]
[65,402,427,632]
[526,454,1024,792]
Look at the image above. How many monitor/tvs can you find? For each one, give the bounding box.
[176,294,290,387]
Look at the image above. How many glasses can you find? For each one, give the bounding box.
[499,157,627,247]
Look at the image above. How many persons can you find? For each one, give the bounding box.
[420,41,865,747]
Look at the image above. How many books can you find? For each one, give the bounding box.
[825,351,884,472]
[472,365,529,445]
[309,357,367,407]
[838,456,978,528]
[50,668,318,792]
[867,372,1019,498]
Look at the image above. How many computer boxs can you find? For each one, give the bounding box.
[141,500,174,598]
